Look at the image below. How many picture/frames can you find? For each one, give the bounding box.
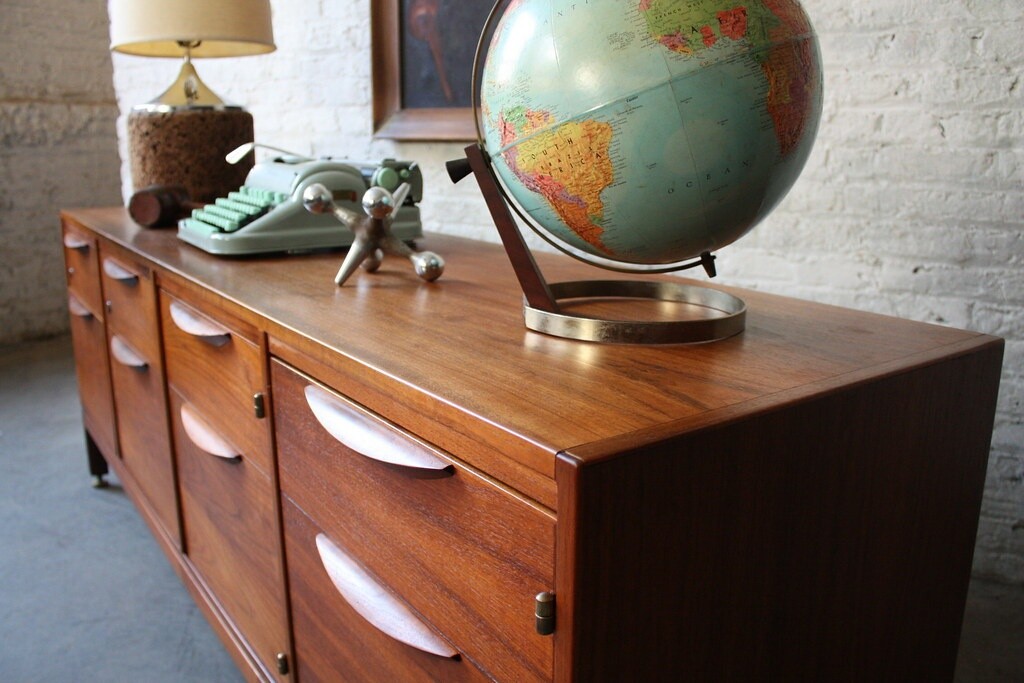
[370,0,501,143]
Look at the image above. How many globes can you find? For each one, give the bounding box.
[446,1,832,347]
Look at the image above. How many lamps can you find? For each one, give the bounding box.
[110,0,278,206]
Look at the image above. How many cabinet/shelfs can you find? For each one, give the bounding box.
[61,209,1006,682]
